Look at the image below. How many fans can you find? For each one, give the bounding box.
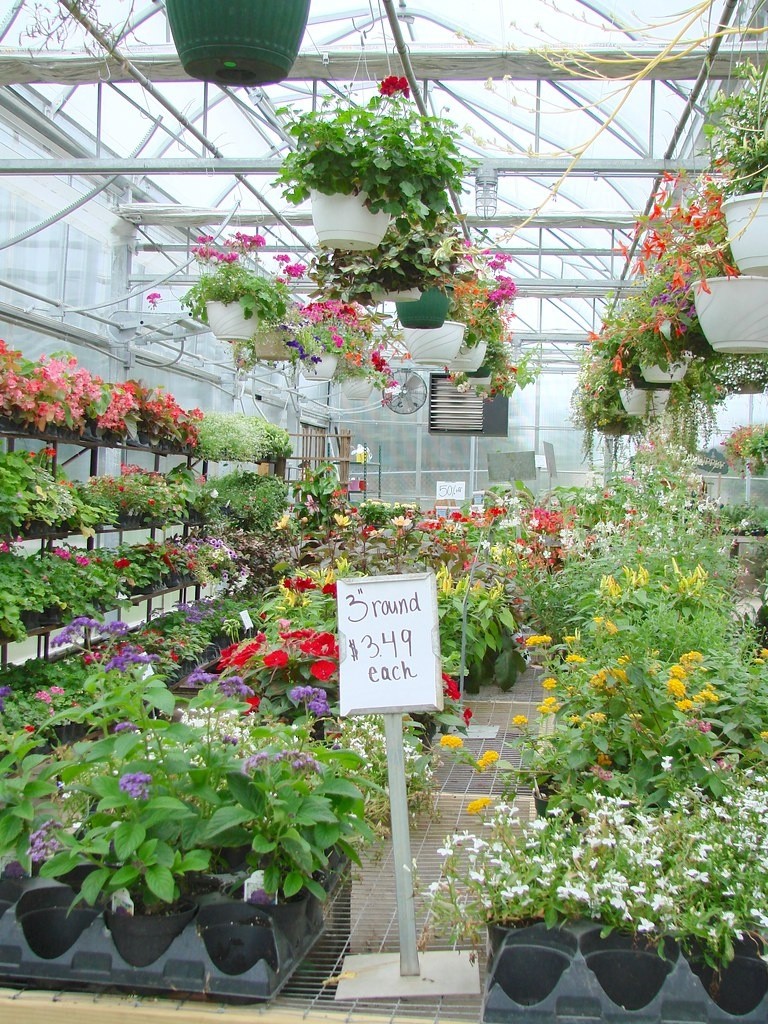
[382,368,427,414]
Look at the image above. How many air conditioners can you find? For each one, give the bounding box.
[427,372,509,437]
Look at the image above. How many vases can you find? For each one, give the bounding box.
[618,379,670,415]
[395,284,453,328]
[105,897,198,966]
[341,375,374,401]
[448,342,488,372]
[300,352,338,381]
[690,274,768,354]
[629,365,672,390]
[165,0,311,87]
[639,351,689,383]
[465,367,493,378]
[310,189,392,250]
[254,332,292,360]
[404,321,466,365]
[206,300,258,340]
[720,192,768,275]
[467,375,492,385]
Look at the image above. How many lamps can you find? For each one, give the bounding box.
[474,167,499,222]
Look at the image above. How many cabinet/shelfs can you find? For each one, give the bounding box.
[0,431,283,674]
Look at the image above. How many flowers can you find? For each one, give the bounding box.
[0,56,768,986]
